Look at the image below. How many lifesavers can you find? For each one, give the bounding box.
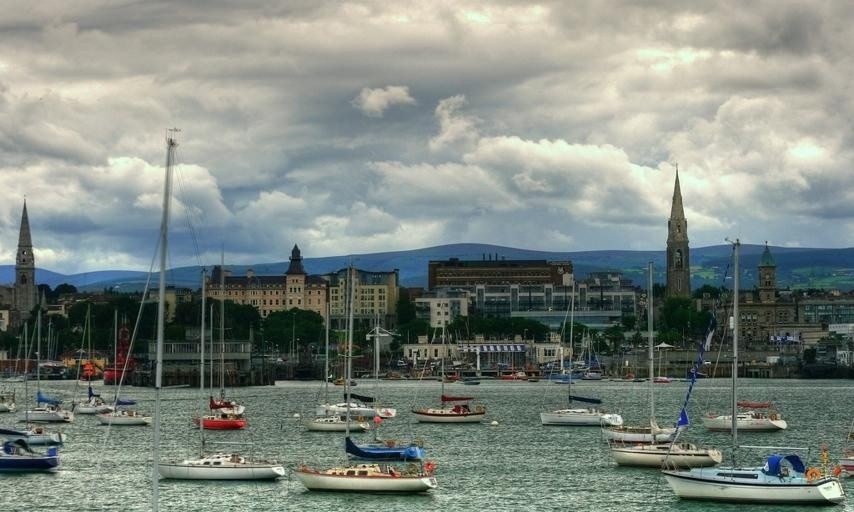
[807,469,820,481]
[424,462,434,471]
